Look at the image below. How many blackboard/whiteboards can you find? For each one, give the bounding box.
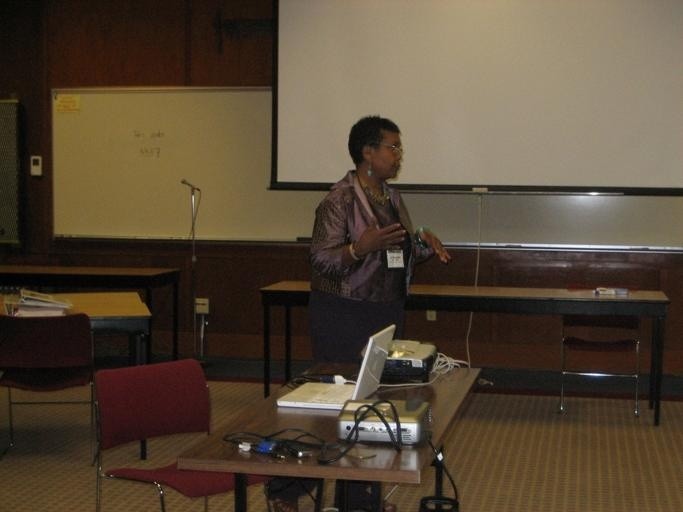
[49,86,683,253]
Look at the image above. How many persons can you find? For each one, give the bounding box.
[305,114,449,512]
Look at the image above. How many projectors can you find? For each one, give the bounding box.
[336,398,432,446]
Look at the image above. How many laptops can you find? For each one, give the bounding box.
[276,321,398,409]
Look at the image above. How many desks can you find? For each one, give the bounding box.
[0,292,152,459]
[178,368,491,512]
[0,264,181,365]
[260,279,669,426]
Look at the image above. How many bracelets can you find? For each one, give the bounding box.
[414,224,431,250]
[349,242,360,261]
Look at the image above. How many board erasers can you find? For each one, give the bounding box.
[297,237,313,242]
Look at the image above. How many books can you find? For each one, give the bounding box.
[4,287,73,317]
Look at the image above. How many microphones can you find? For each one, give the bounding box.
[180,178,201,192]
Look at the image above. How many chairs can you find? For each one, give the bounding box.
[95,359,278,512]
[0,314,101,465]
[559,273,641,416]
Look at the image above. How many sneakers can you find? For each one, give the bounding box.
[335,493,398,512]
[263,480,300,512]
